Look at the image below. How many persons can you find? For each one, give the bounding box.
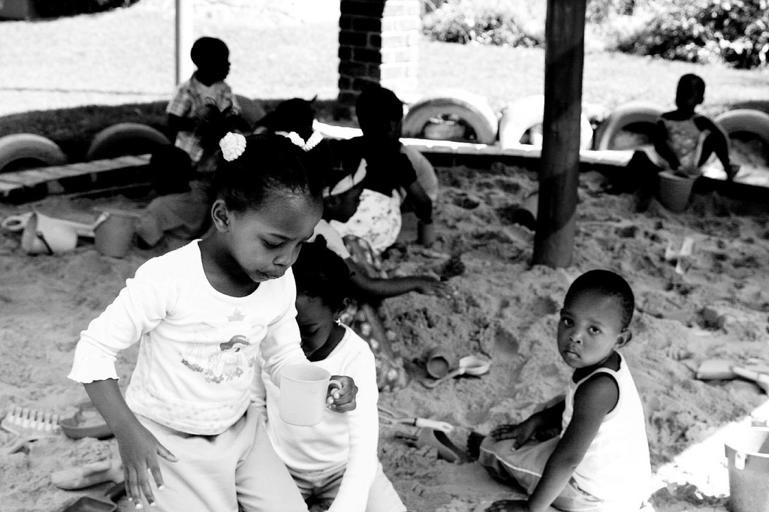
[262,244,408,511]
[636,73,741,214]
[467,269,652,511]
[67,129,359,511]
[165,35,254,182]
[271,85,466,328]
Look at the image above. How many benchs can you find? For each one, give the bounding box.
[1,127,764,209]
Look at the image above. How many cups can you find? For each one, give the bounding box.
[417,220,435,246]
[279,365,341,426]
[427,347,453,377]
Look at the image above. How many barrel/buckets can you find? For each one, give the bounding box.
[658,168,692,214]
